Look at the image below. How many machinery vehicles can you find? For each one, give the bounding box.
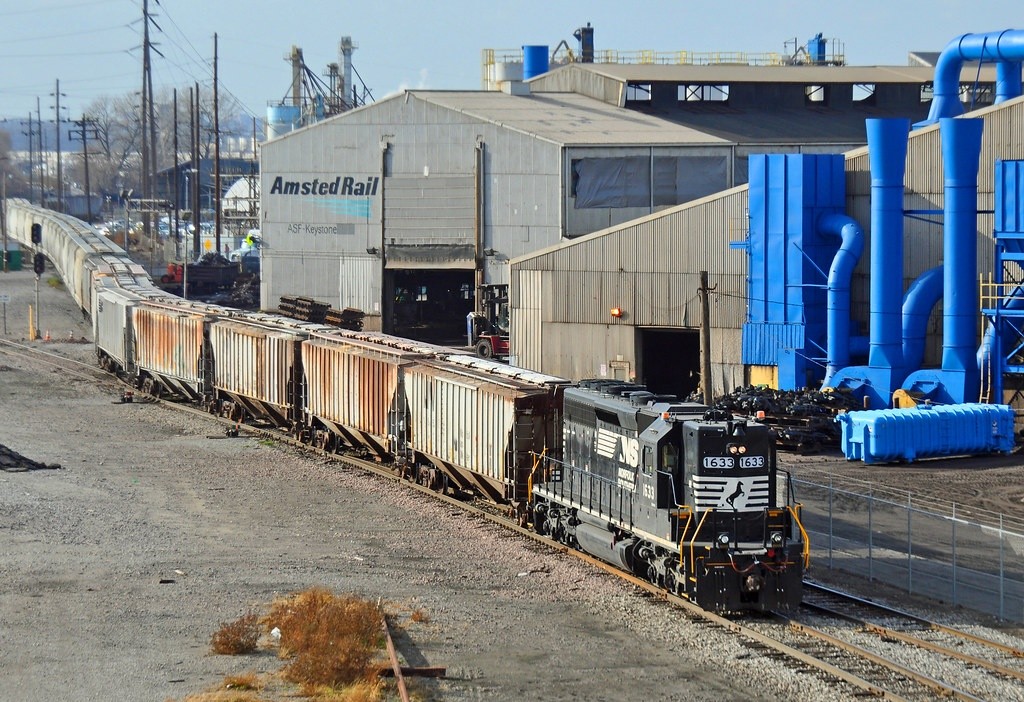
[466,283,510,360]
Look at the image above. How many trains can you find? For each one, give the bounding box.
[0,198,810,618]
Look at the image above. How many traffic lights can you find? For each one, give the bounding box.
[34,253,45,274]
[31,223,42,243]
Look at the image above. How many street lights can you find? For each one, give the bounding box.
[120,190,129,253]
[182,210,193,299]
